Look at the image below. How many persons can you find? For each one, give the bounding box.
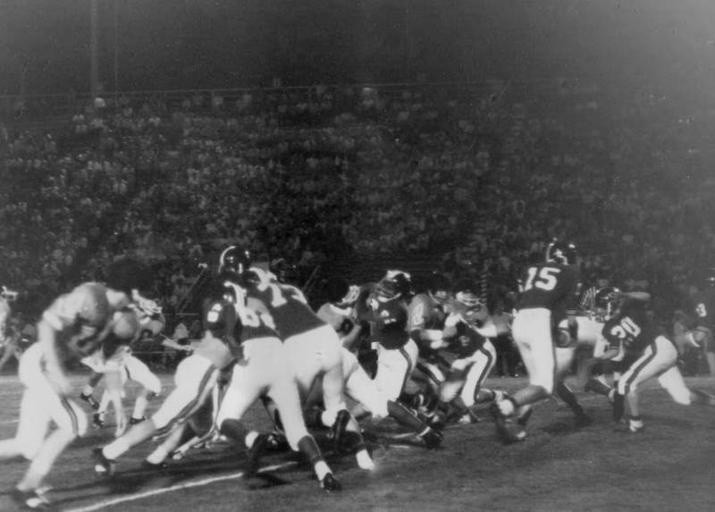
[1,236,715,512]
[1,68,715,374]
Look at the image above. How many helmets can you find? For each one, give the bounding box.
[125,288,165,345]
[376,269,412,302]
[428,275,483,317]
[595,286,625,322]
[544,236,579,266]
[219,246,251,278]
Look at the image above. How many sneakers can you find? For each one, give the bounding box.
[694,390,715,406]
[609,390,624,423]
[326,409,349,457]
[90,447,116,483]
[410,391,455,449]
[490,389,533,442]
[243,433,271,476]
[140,459,185,477]
[319,473,342,492]
[129,417,145,425]
[9,483,54,512]
[79,393,99,409]
[92,411,105,428]
